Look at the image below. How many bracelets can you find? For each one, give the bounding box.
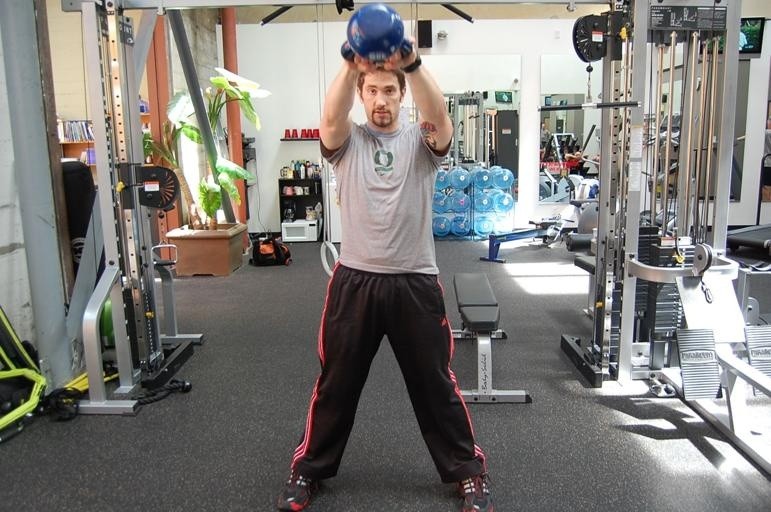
[400,55,422,72]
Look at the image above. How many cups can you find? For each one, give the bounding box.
[285,128,321,139]
[282,186,309,195]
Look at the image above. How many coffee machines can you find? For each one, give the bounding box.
[282,200,299,223]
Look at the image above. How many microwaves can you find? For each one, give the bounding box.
[281,219,316,242]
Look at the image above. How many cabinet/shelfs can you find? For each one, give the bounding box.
[279,177,323,236]
[56,139,96,170]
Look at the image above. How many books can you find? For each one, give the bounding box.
[64,120,96,165]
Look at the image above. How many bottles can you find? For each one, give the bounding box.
[279,160,320,180]
[306,201,323,220]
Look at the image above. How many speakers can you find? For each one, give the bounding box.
[418,20,432,48]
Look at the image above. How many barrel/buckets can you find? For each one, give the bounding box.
[432,166,514,237]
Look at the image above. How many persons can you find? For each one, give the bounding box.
[540,124,550,148]
[761,118,771,201]
[277,40,494,512]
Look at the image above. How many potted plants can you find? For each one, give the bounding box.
[138,63,271,282]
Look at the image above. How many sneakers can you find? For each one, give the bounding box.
[458,474,493,511]
[275,469,320,511]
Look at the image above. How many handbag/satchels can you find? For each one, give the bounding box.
[253,239,290,266]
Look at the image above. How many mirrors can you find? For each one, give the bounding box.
[418,90,520,174]
[541,94,584,160]
[34,0,123,312]
[648,56,750,202]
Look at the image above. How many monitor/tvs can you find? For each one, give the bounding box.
[698,16,766,62]
